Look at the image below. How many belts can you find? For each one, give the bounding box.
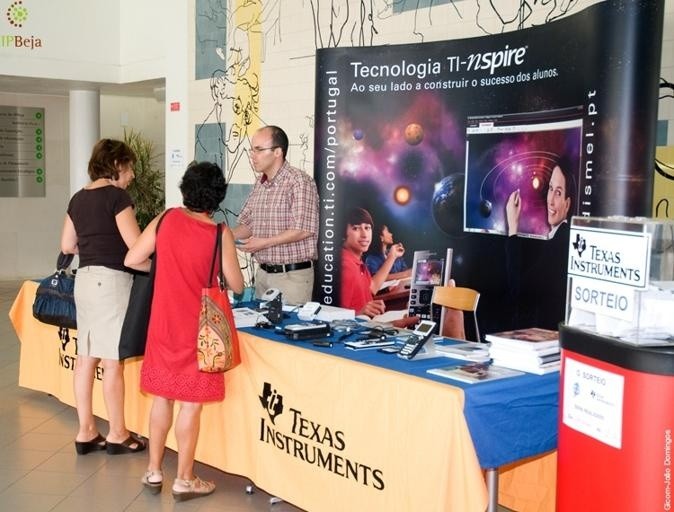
[260,261,311,273]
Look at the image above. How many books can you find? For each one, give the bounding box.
[422,327,561,376]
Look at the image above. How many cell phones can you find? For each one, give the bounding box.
[397,319,438,359]
[514,188,522,205]
[407,248,452,336]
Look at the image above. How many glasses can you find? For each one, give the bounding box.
[249,146,279,154]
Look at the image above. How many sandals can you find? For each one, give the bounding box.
[141,470,163,496]
[172,474,216,503]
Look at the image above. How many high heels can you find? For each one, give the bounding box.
[105,432,146,455]
[74,432,107,456]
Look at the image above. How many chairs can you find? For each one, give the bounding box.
[428,283,484,344]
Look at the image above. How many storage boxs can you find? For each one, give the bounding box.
[563,211,674,351]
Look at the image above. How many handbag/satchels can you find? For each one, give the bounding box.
[117,274,159,362]
[32,249,79,330]
[199,285,242,374]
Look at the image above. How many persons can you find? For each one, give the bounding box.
[341,206,406,319]
[366,223,407,276]
[61,139,146,455]
[124,160,244,503]
[230,126,320,306]
[506,159,577,241]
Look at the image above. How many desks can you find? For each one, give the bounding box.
[5,267,560,512]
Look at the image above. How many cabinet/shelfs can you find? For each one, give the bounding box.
[554,321,673,512]
